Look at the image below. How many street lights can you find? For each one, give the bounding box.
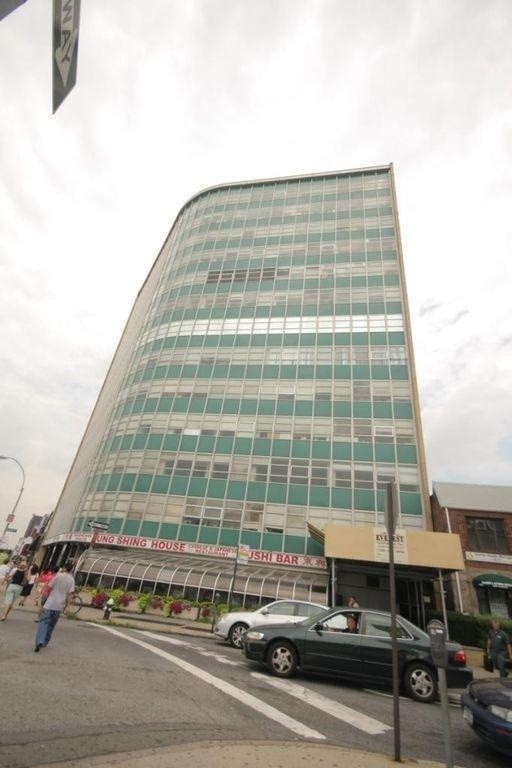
[1,454,25,543]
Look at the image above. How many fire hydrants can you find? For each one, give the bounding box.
[102,598,114,620]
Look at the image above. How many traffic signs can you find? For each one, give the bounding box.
[88,521,109,530]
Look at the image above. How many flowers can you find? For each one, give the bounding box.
[88,588,228,624]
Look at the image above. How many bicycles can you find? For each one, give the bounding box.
[67,591,82,615]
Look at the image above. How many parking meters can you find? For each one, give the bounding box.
[425,619,453,768]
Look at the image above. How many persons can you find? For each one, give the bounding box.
[349,596,359,607]
[34,562,75,652]
[486,617,512,677]
[0,553,60,622]
[328,613,359,634]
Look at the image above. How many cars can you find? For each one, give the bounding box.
[241,606,473,704]
[213,599,350,650]
[461,677,511,759]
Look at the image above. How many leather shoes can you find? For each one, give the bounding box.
[35,643,42,651]
